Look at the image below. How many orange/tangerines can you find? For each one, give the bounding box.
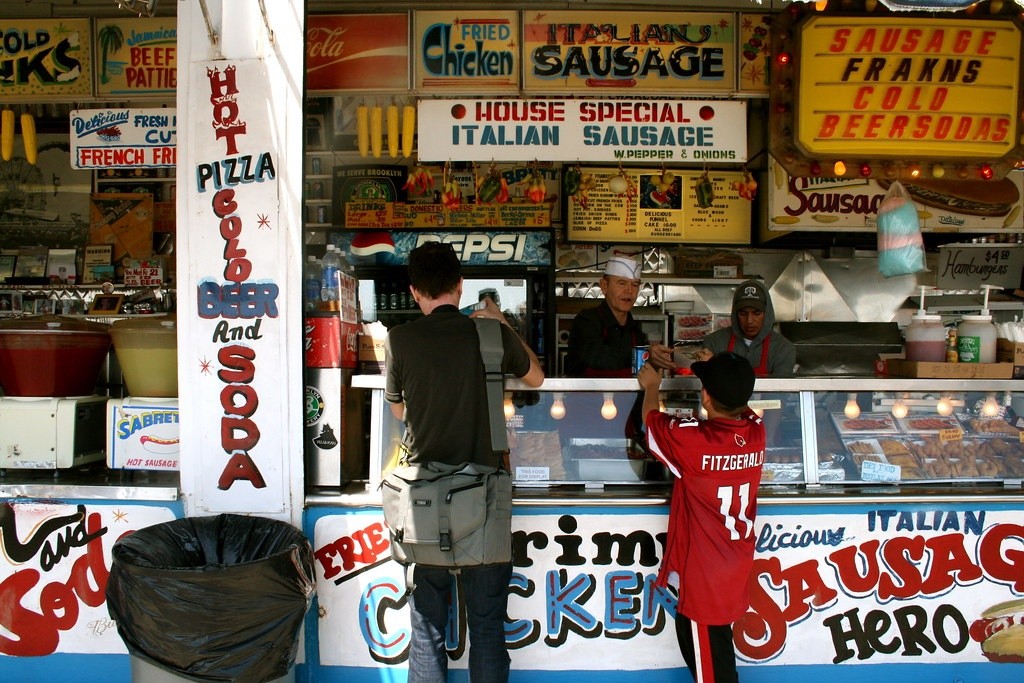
[651,173,675,192]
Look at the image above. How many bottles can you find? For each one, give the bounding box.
[305,245,359,312]
[904,315,946,363]
[957,314,997,363]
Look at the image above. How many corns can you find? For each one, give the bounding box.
[21,114,37,165]
[1,109,15,161]
[357,106,415,158]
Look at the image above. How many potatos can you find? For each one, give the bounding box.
[580,174,596,191]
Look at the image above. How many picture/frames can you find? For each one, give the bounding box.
[89,294,125,315]
[81,242,115,284]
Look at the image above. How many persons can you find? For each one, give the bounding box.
[563,254,673,441]
[385,241,546,683]
[700,280,796,455]
[637,352,766,683]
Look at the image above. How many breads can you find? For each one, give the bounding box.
[850,419,1024,479]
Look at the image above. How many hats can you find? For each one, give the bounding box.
[690,351,756,409]
[732,280,767,316]
[603,257,642,280]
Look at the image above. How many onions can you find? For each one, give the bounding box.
[732,179,758,191]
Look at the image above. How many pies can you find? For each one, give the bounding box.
[843,418,956,429]
[678,316,708,339]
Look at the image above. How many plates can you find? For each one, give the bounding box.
[830,412,1024,479]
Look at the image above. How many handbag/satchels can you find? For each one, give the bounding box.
[375,460,512,567]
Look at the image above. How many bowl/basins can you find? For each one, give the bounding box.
[109,319,178,398]
[0,312,109,398]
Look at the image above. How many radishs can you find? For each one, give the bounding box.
[609,177,628,195]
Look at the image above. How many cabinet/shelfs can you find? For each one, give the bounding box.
[556,314,669,379]
[95,149,419,228]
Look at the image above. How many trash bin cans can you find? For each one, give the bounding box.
[110,512,317,683]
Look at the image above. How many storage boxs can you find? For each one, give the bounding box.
[996,338,1024,380]
[887,358,1014,379]
[304,317,361,367]
[356,333,387,373]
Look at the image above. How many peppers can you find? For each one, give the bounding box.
[626,179,638,199]
[403,168,590,209]
[662,185,676,202]
[739,181,758,201]
[695,180,713,209]
